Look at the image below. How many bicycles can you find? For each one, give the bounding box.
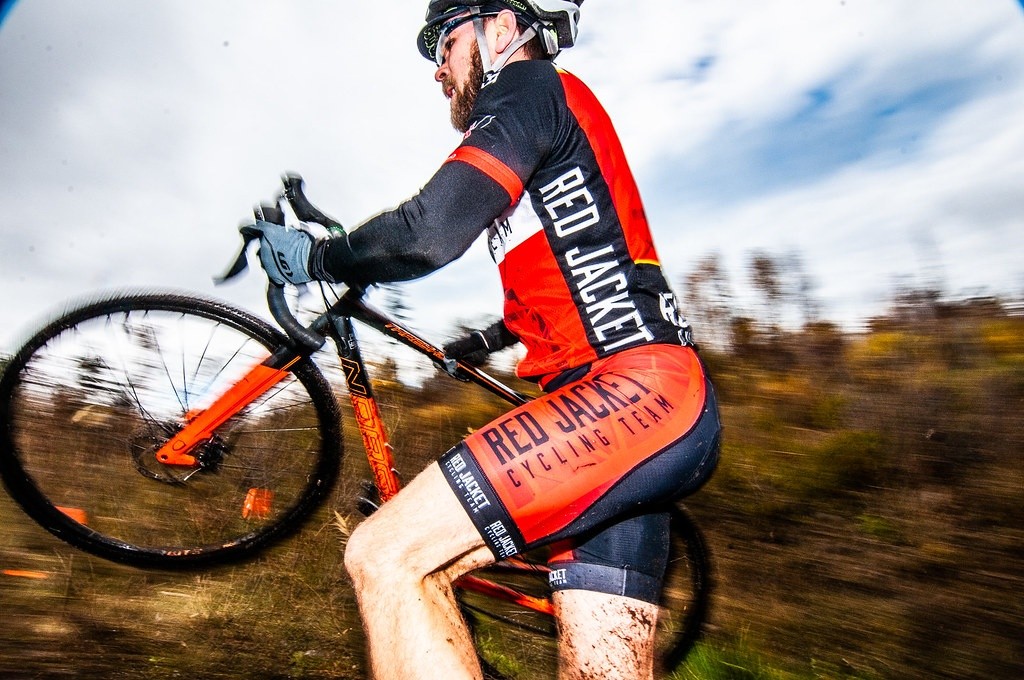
[0,175,713,680]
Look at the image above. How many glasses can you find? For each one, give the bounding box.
[434,11,522,65]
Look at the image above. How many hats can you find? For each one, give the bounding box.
[416,6,536,62]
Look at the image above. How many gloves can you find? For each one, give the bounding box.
[432,333,492,385]
[240,218,312,287]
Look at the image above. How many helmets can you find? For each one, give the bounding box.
[426,0,584,49]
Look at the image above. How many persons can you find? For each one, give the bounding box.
[238,0,723,680]
